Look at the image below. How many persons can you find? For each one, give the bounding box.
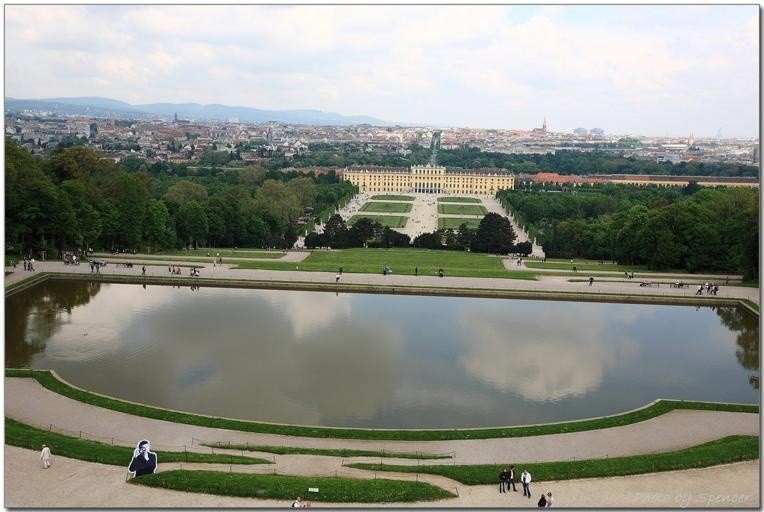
[293,496,304,507]
[10,240,728,296]
[303,501,312,507]
[40,443,51,469]
[537,493,546,507]
[499,467,508,494]
[128,440,158,478]
[507,464,518,492]
[546,492,553,507]
[521,468,532,499]
